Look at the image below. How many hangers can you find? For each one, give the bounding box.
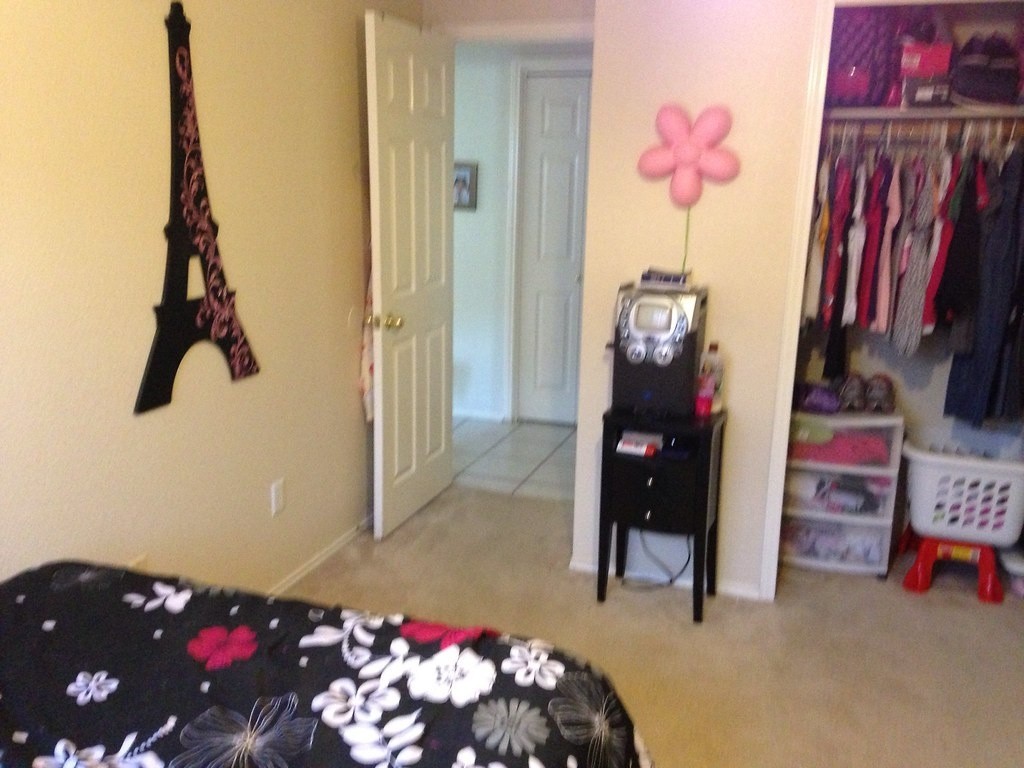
[822,118,1019,163]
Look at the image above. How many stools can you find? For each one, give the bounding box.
[900,536,1005,603]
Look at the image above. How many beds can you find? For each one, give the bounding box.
[0,557,653,768]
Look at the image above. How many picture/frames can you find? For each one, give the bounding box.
[453,162,478,210]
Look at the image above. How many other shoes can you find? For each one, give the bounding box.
[839,373,863,412]
[866,374,895,411]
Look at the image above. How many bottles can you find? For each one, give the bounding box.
[702,343,725,415]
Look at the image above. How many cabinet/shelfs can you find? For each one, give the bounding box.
[595,408,729,624]
[778,411,904,583]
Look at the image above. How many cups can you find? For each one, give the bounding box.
[695,375,716,415]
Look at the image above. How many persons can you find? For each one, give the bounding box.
[453,171,469,206]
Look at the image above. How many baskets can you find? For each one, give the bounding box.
[901,430,1024,547]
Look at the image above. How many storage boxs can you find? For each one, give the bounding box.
[898,427,1024,549]
[895,41,952,111]
